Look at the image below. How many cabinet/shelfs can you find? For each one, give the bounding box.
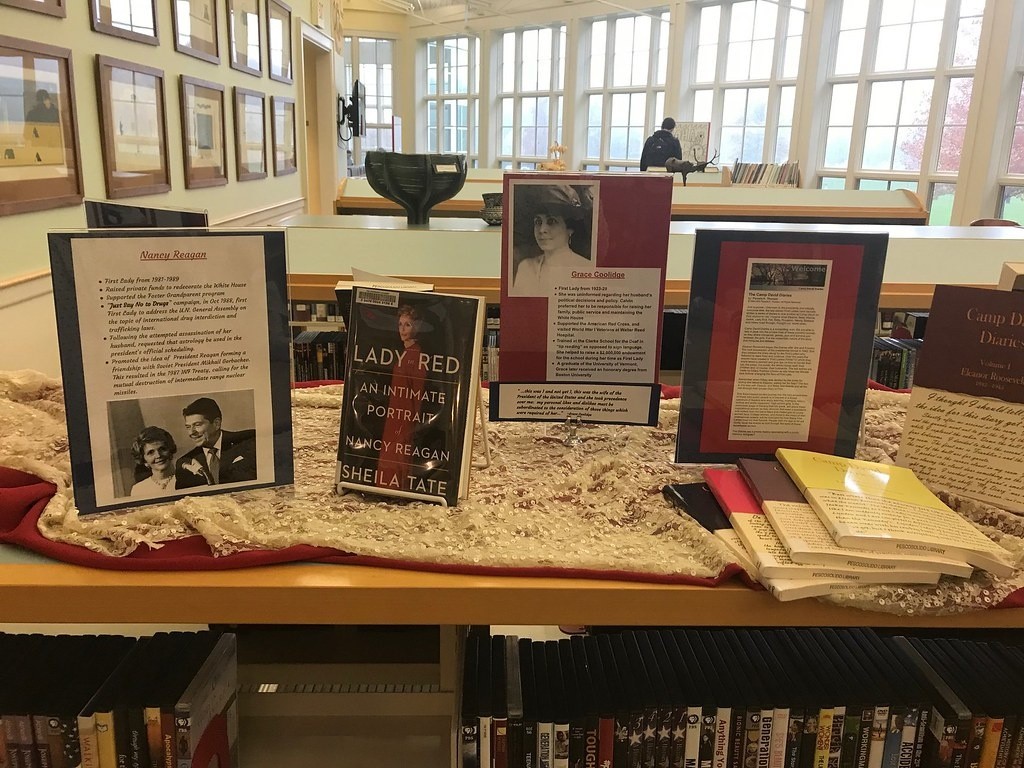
[0,175,1024,768]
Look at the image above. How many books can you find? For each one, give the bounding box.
[0,632,238,768]
[662,448,1014,601]
[870,311,929,390]
[458,629,1024,768]
[730,160,800,184]
[292,299,499,381]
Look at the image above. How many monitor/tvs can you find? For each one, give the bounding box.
[351,80,367,136]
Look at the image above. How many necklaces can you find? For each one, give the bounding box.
[151,472,173,491]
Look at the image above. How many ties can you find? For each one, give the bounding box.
[209,448,220,484]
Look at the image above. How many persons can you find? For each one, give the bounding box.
[512,182,592,298]
[376,303,435,490]
[125,397,254,495]
[25,89,60,146]
[640,117,682,172]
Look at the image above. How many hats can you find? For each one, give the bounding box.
[526,185,589,220]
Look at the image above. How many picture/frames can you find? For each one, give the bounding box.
[0,0,297,218]
